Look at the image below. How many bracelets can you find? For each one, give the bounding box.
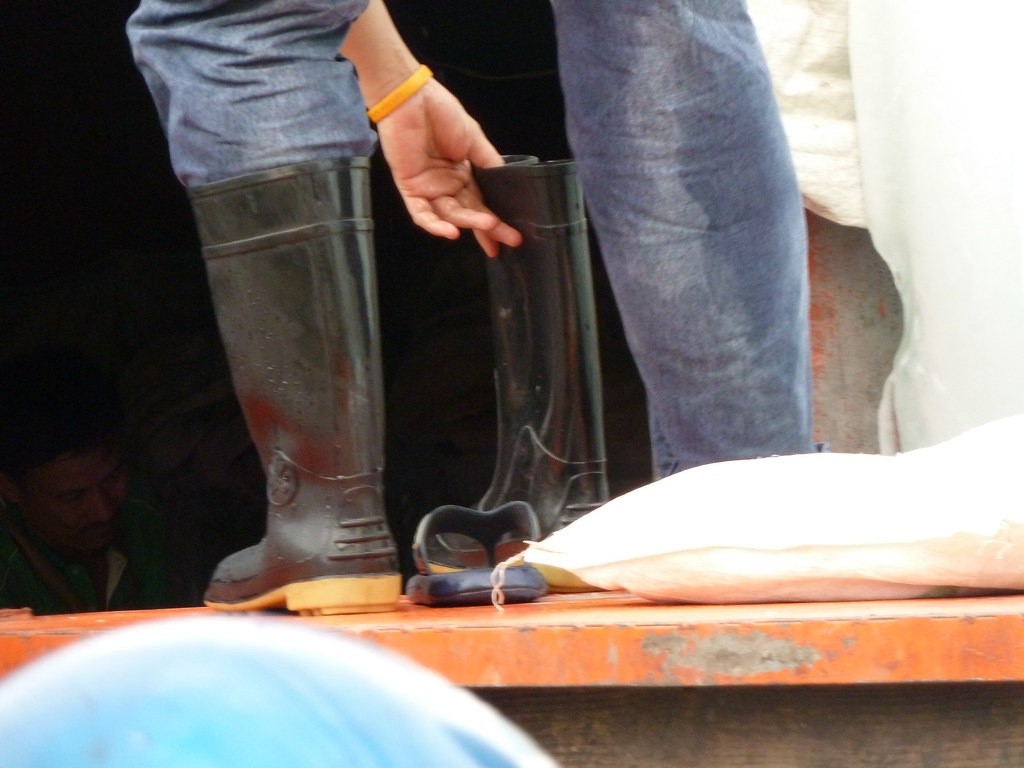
[361,63,435,123]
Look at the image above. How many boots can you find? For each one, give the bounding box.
[186,156,400,616]
[419,155,611,574]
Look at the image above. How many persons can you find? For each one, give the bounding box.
[0,357,171,610]
[122,0,814,615]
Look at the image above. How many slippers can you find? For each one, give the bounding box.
[406,501,546,606]
